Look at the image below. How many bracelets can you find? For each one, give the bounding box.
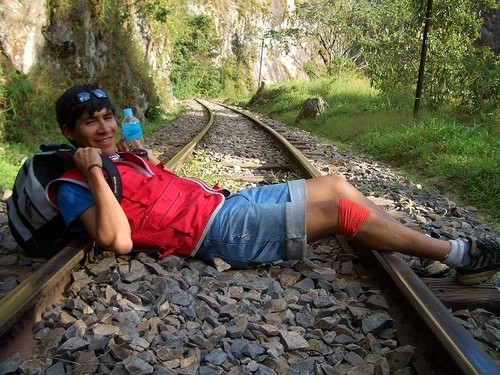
[83,165,103,178]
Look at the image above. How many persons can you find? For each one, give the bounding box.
[46,84,500,286]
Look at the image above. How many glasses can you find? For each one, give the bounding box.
[67,89,108,117]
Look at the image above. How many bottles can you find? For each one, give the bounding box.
[121,108,149,161]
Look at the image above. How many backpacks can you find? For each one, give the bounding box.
[6,143,149,258]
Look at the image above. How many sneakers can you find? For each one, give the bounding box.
[454,235,500,285]
[428,231,455,275]
